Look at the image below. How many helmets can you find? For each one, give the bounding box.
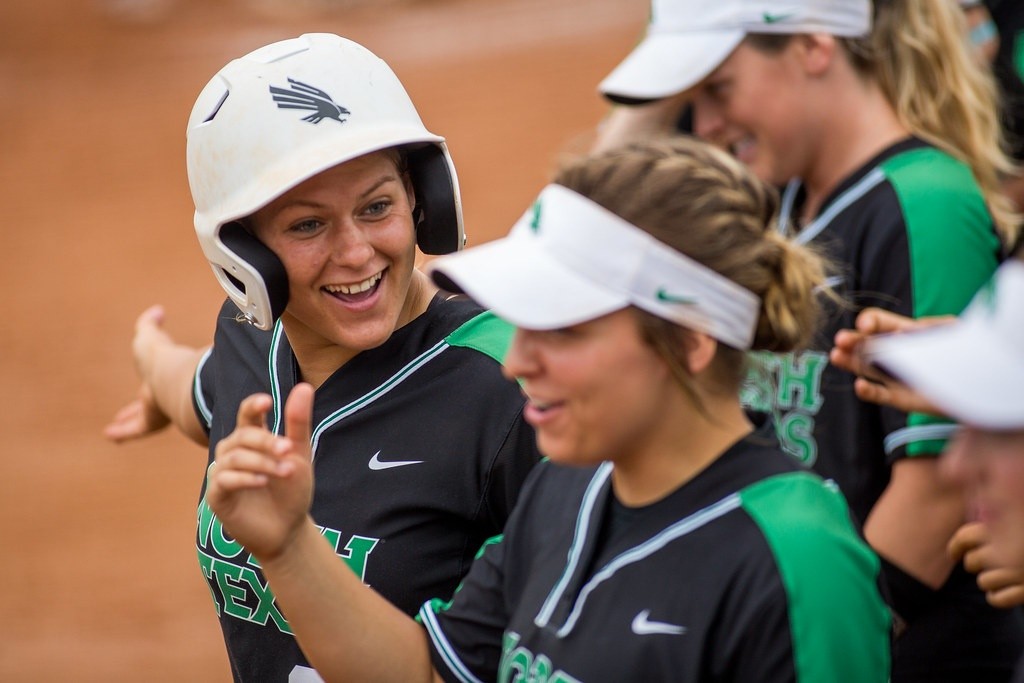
[184,33,467,330]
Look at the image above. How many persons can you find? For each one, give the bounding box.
[588,0,1024,683]
[828,0,1024,611]
[202,130,900,683]
[103,29,545,682]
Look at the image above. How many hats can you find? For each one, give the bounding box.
[862,260,1023,433]
[426,181,762,350]
[597,1,871,106]
